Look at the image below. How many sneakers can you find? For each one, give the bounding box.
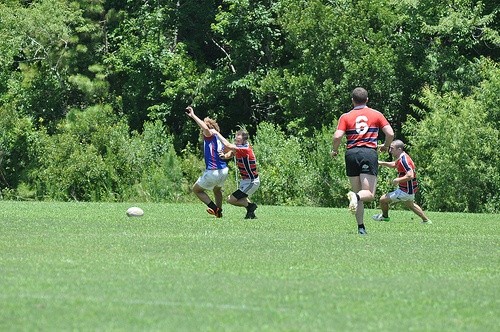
[346,191,359,215]
[245,202,257,219]
[358,228,366,234]
[207,207,222,215]
[372,213,390,221]
[420,220,432,224]
[215,207,222,218]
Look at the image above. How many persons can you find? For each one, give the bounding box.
[186,107,228,218]
[331,87,394,234]
[373,139,433,224]
[211,128,260,219]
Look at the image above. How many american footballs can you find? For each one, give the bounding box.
[126,206,144,217]
